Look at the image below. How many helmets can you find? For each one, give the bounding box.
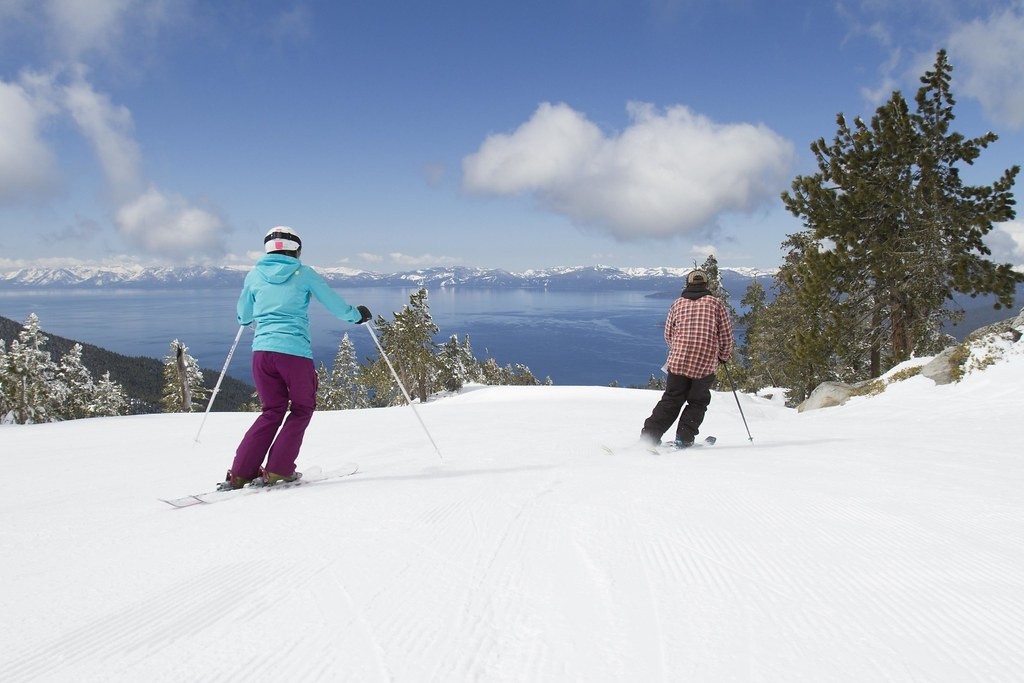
[263,225,302,253]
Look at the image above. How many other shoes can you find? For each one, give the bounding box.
[262,469,302,485]
[640,429,662,447]
[675,433,693,448]
[227,466,263,488]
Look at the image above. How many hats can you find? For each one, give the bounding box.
[687,270,708,285]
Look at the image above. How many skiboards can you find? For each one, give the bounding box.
[157,462,359,509]
[602,435,717,457]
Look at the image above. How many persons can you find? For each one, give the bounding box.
[641,272,736,449]
[216,226,373,491]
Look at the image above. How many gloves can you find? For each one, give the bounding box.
[355,305,373,324]
